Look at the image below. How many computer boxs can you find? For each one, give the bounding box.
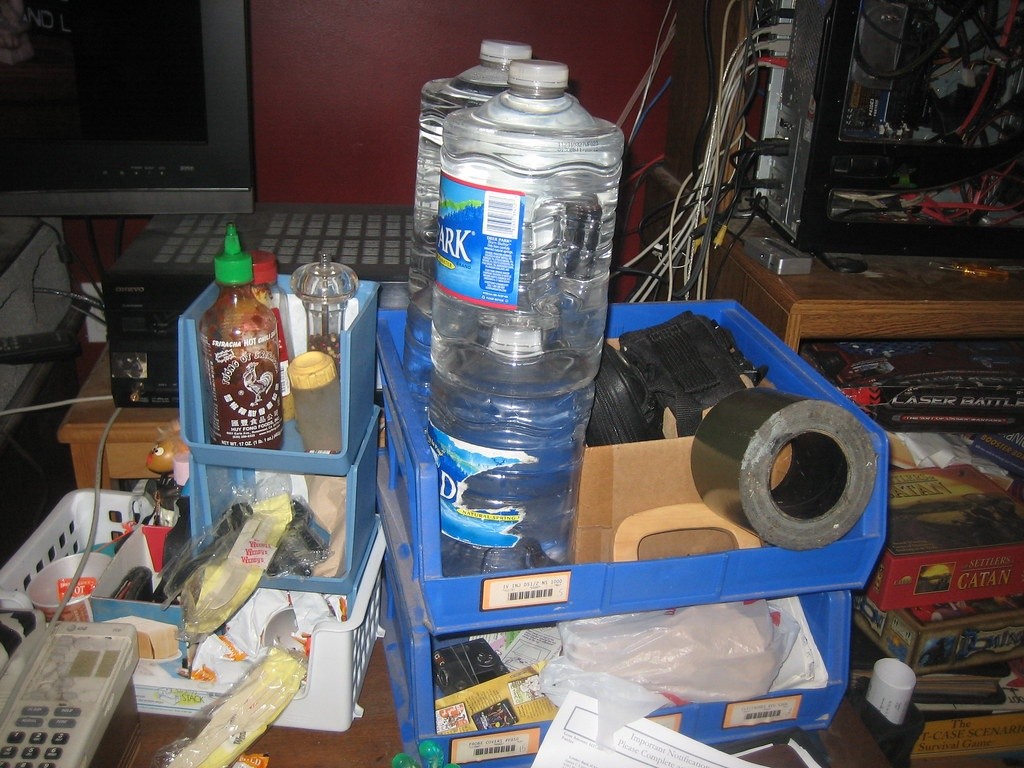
[751,0,1024,260]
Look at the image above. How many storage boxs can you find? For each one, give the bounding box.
[381,488,853,768]
[190,406,383,596]
[179,275,379,478]
[573,337,798,566]
[0,489,386,733]
[376,298,890,636]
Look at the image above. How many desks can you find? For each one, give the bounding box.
[643,156,1024,352]
[57,337,182,490]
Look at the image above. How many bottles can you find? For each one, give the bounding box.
[285,350,344,458]
[244,247,301,423]
[402,36,627,550]
[195,221,285,456]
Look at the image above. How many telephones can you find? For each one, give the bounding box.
[0,609,139,768]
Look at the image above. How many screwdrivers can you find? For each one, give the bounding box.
[930,260,1011,283]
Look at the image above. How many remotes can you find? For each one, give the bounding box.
[0,330,83,365]
[0,619,141,768]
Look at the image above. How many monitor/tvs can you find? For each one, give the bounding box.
[0,0,256,215]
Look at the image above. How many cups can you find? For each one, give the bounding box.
[23,549,115,624]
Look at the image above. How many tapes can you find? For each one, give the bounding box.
[692,387,876,550]
